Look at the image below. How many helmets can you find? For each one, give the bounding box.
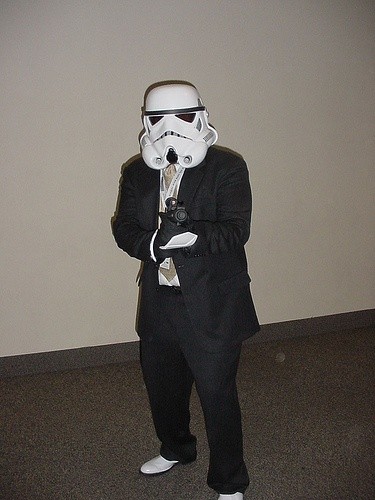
[139,83,217,169]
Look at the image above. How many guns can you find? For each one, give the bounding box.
[166,205,187,226]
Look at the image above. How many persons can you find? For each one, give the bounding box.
[111,83,261,500]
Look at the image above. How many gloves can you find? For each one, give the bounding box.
[158,212,199,257]
[152,230,176,264]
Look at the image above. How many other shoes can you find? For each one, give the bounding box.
[217,491,243,500]
[140,455,179,475]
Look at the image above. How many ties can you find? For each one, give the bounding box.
[159,163,178,282]
